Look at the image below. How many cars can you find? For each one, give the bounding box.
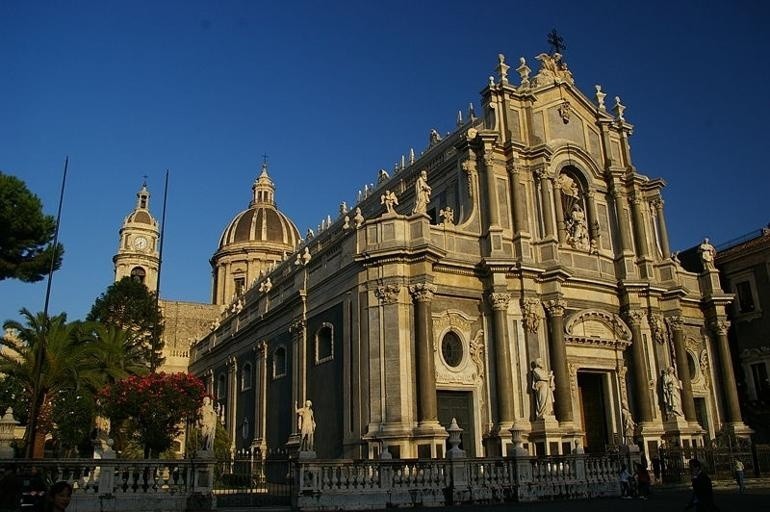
[0,437,88,512]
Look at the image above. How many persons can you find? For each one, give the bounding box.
[734,459,744,494]
[652,455,660,482]
[414,170,431,214]
[663,367,684,417]
[48,482,72,512]
[698,238,716,270]
[620,463,650,498]
[295,399,316,452]
[572,204,589,240]
[196,397,217,454]
[532,358,555,417]
[689,459,713,504]
[98,439,116,494]
